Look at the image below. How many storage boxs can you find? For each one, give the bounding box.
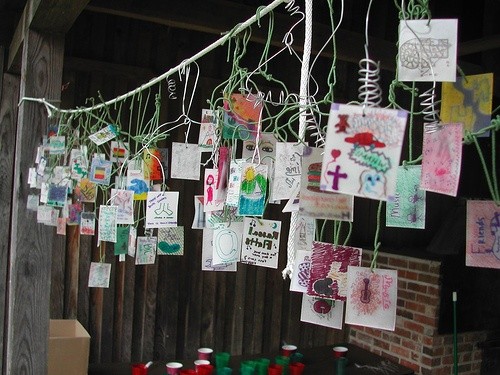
[48,318,92,375]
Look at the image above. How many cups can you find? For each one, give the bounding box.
[165,362,183,375]
[332,346,348,375]
[130,363,149,375]
[179,345,305,375]
